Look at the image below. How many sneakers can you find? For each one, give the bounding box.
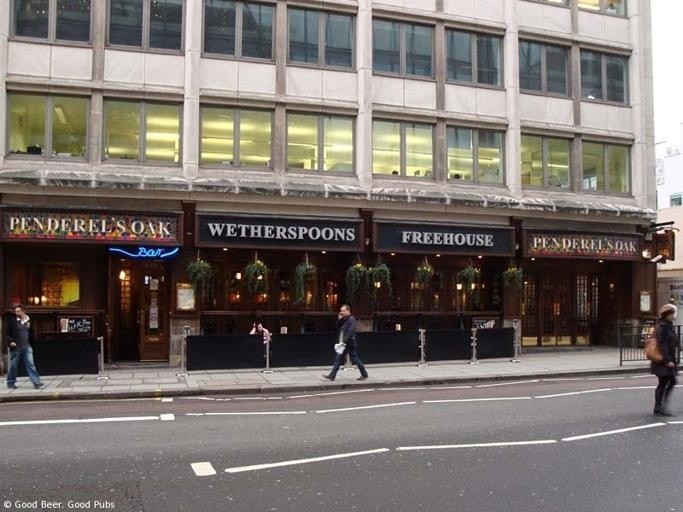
[34,381,45,389]
[8,384,17,389]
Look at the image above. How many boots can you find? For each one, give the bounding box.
[654,387,675,417]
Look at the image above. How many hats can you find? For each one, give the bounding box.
[659,302,677,319]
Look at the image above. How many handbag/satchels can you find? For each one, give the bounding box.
[643,324,663,364]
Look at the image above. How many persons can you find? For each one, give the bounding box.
[249,321,270,336]
[5,304,44,389]
[644,303,683,417]
[321,304,369,381]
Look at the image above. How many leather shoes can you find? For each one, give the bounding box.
[322,373,335,381]
[357,374,369,381]
